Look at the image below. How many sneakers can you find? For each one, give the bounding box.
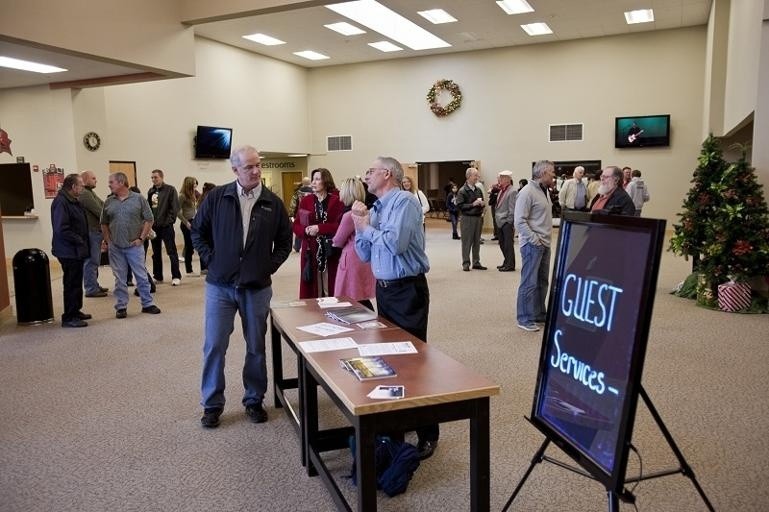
[517,321,539,332]
[184,269,209,277]
[116,309,127,318]
[201,407,224,426]
[246,403,268,423]
[127,278,181,314]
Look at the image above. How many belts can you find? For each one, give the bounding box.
[377,281,407,288]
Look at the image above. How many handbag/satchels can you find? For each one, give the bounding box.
[322,236,341,258]
[349,436,421,498]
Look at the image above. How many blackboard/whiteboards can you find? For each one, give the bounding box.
[0,163,39,219]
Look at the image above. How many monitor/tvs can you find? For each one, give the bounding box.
[0,163,34,216]
[615,114,670,148]
[196,125,232,158]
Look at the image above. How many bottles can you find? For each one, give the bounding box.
[374,438,393,477]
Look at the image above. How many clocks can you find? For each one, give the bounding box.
[84,132,101,152]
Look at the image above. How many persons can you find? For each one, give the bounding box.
[330,177,378,314]
[50,174,92,327]
[190,144,292,428]
[513,161,556,332]
[78,169,109,298]
[125,169,217,298]
[350,155,429,343]
[99,171,160,318]
[288,176,313,253]
[400,175,430,232]
[627,121,644,140]
[291,168,346,298]
[436,161,652,277]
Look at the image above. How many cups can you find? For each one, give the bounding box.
[477,198,483,203]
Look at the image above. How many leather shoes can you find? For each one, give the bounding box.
[62,312,91,327]
[86,288,109,297]
[416,440,437,459]
[463,265,515,272]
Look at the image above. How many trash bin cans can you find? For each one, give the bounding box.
[13,248,55,328]
